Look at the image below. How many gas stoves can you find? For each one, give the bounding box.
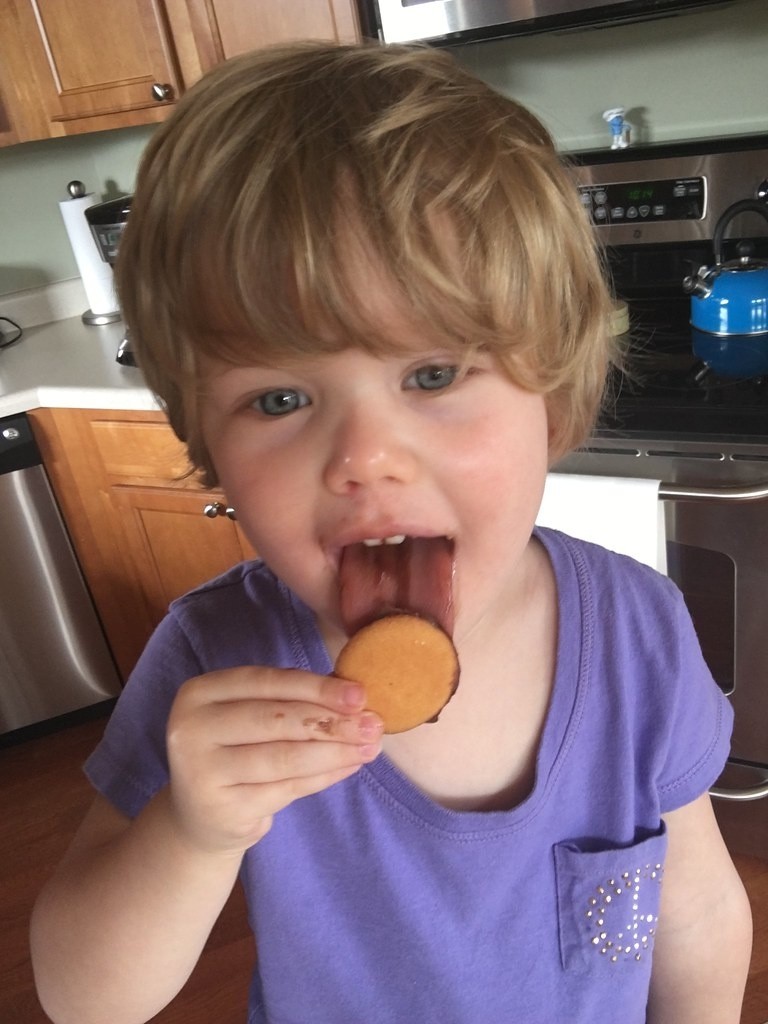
[522,135,768,443]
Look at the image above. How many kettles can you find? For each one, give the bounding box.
[680,199,767,389]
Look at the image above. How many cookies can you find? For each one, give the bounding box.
[331,612,461,735]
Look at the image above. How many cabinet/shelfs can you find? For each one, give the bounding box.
[1,0,380,150]
[25,386,267,693]
[524,125,768,859]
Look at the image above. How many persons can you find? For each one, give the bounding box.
[28,44,752,1024]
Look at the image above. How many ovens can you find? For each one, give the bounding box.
[539,438,768,866]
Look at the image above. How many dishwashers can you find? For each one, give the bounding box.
[0,409,126,736]
